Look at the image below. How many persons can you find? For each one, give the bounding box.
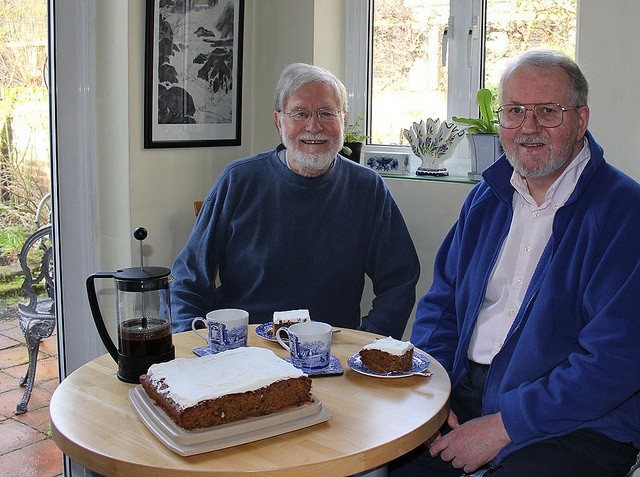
[392,49,639,477]
[161,63,420,339]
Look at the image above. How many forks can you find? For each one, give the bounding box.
[367,365,433,378]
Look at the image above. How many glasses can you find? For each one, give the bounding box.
[277,107,344,123]
[493,102,581,129]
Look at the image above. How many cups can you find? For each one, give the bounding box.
[276,321,333,370]
[191,308,250,353]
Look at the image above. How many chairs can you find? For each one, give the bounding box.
[12,223,57,416]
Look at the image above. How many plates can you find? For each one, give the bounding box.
[193,346,214,357]
[347,350,429,379]
[255,320,317,342]
[284,354,344,378]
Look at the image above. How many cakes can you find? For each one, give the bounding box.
[359,336,415,373]
[270,310,310,338]
[139,347,312,429]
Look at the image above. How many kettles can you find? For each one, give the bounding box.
[85,226,175,385]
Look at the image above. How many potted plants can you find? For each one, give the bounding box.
[450,87,503,183]
[341,114,373,165]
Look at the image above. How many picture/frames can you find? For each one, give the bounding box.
[141,0,246,151]
